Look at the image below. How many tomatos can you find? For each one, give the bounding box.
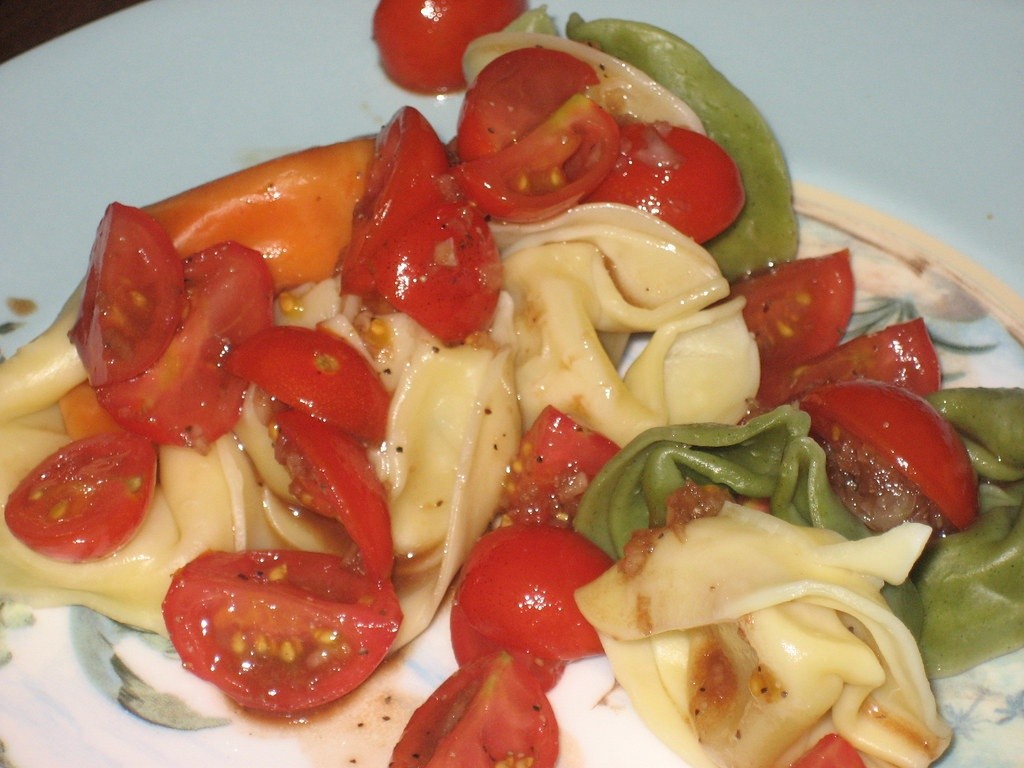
[6,0,973,768]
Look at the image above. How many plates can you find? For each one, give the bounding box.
[0,0,1024,768]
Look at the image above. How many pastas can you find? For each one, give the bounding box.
[0,201,958,768]
